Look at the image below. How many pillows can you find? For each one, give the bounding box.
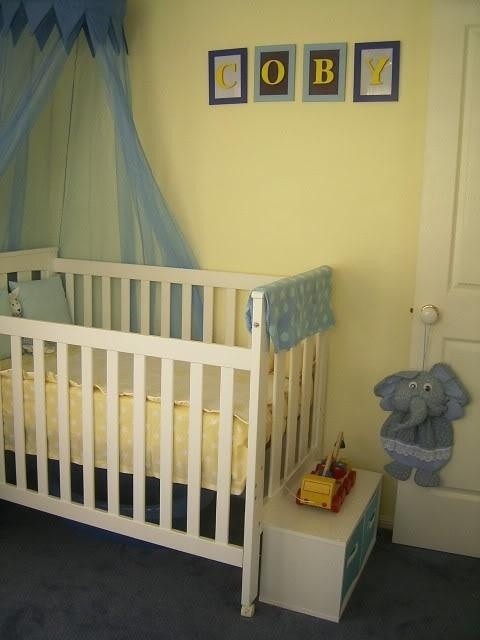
[8,275,73,346]
[0,289,25,360]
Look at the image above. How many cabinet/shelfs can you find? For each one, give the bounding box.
[258,463,384,622]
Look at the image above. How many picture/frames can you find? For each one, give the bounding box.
[302,42,346,102]
[253,43,295,101]
[352,41,399,102]
[208,48,247,105]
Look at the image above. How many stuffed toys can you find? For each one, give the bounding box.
[373,363,469,488]
[8,287,56,354]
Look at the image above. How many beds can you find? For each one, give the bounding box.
[1,246,333,617]
